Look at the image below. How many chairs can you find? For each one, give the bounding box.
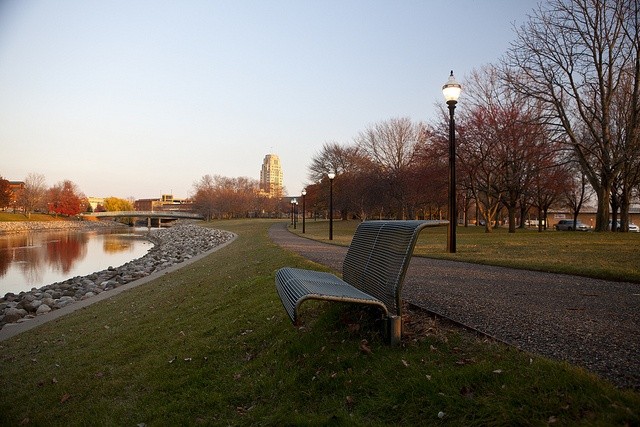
[274,220,450,345]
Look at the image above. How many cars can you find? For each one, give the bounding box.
[524,220,537,226]
[556,219,588,232]
[609,219,639,232]
[475,220,486,226]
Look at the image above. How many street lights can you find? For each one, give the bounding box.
[291,200,293,225]
[293,198,296,229]
[302,188,307,233]
[328,166,336,239]
[443,70,462,253]
[296,202,298,222]
